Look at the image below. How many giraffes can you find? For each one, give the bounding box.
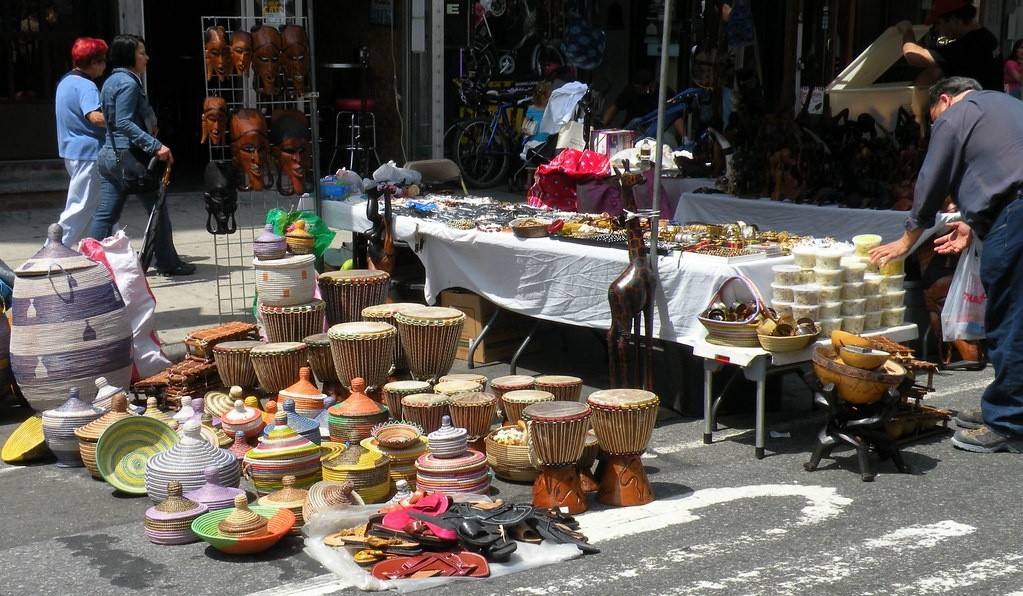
[611,159,658,391]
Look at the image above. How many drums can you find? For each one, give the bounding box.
[261,297,326,348]
[400,392,450,436]
[302,332,351,403]
[519,399,593,515]
[361,302,426,383]
[328,320,398,393]
[212,339,265,412]
[316,268,391,328]
[396,305,468,380]
[250,342,309,402]
[577,431,598,493]
[534,376,583,402]
[433,380,484,428]
[586,388,660,510]
[490,373,536,430]
[446,391,499,456]
[439,373,488,392]
[502,388,556,431]
[383,380,433,424]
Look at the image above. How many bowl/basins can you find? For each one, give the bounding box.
[812,266,845,287]
[882,274,906,292]
[877,258,904,276]
[757,334,812,352]
[842,315,867,335]
[863,276,883,296]
[793,246,816,268]
[819,302,842,319]
[841,299,866,315]
[864,310,882,331]
[883,291,906,308]
[840,346,890,369]
[821,318,842,338]
[865,294,883,312]
[817,285,843,302]
[839,247,867,283]
[852,234,883,257]
[812,347,907,404]
[816,249,844,270]
[771,298,821,323]
[770,282,818,306]
[773,265,817,287]
[881,305,907,327]
[841,281,865,299]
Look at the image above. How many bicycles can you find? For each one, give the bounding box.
[444,0,733,192]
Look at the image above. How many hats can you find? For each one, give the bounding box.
[925,0,973,24]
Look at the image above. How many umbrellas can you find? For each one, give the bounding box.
[136,154,168,274]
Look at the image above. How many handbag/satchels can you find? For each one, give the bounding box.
[942,234,987,340]
[116,157,160,195]
[524,120,539,135]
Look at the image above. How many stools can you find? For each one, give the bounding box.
[327,95,385,176]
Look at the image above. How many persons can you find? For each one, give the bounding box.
[867,0,1023,453]
[517,74,692,193]
[45,34,193,275]
[201,25,311,195]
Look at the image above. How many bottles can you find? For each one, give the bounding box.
[640,140,651,171]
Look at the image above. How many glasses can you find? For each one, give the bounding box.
[930,96,941,130]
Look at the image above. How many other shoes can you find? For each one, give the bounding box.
[525,185,533,189]
[156,262,197,276]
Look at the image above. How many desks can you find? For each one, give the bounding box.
[693,321,917,460]
[298,183,862,431]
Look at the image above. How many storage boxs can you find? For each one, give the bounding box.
[770,229,908,339]
[252,217,318,307]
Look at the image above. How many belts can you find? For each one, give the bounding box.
[995,188,1023,213]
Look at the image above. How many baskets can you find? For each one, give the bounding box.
[699,275,770,347]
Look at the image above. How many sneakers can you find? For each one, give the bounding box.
[955,408,985,429]
[950,425,1023,453]
